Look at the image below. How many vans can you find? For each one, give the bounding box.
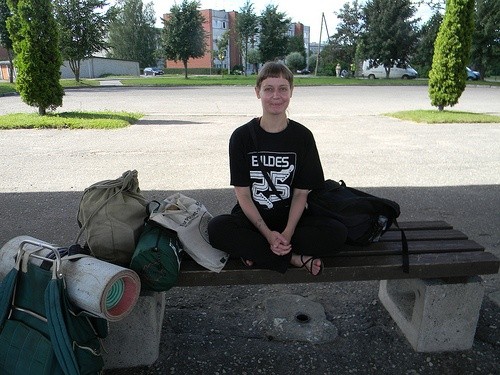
[362,58,418,80]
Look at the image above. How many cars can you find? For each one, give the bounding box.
[466,67,480,81]
[144,67,164,76]
[297,68,308,75]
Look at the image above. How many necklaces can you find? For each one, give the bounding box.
[259,117,288,127]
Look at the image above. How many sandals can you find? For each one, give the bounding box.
[240,255,255,267]
[292,253,326,277]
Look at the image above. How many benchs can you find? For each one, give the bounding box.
[98,219,500,369]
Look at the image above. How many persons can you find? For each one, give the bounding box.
[208,62,326,279]
[336,63,341,77]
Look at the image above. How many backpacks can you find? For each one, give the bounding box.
[310,176,402,247]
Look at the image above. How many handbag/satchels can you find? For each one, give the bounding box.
[77,169,147,267]
[149,192,232,274]
[130,227,188,290]
